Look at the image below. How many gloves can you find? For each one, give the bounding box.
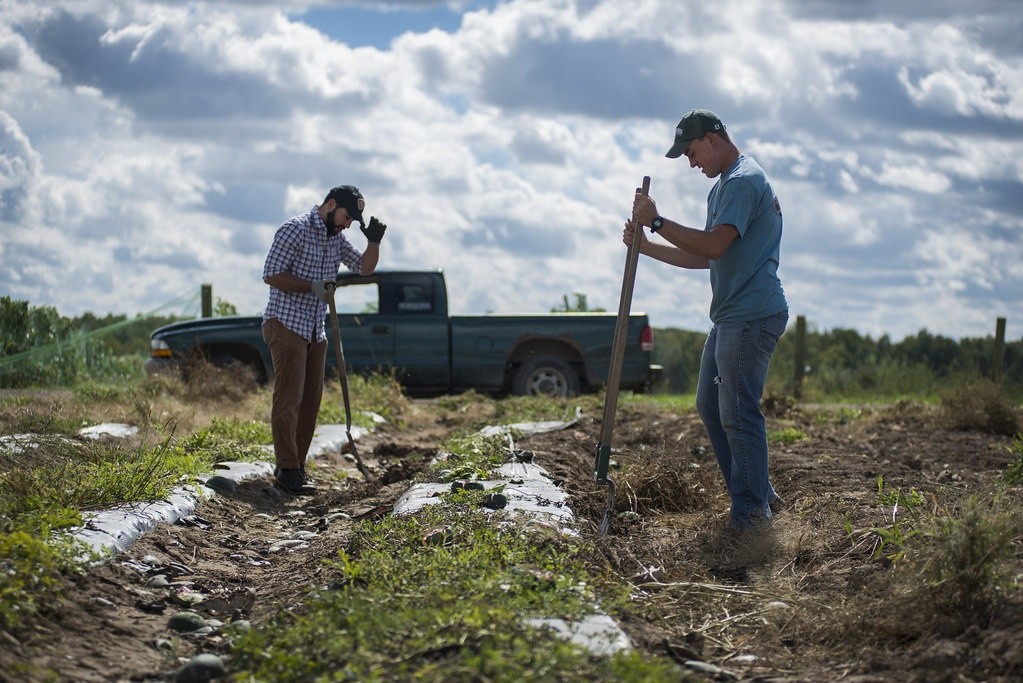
[360,217,386,246]
[311,280,336,303]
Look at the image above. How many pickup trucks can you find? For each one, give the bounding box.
[144,268,664,401]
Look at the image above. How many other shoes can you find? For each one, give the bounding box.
[769,497,786,517]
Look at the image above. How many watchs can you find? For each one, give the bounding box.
[651,217,663,233]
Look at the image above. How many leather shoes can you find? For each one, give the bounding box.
[274,466,316,483]
[273,470,317,495]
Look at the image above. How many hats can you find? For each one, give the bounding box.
[665,109,722,159]
[331,185,364,225]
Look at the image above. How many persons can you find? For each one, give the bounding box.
[623,108,788,571]
[262,185,387,495]
